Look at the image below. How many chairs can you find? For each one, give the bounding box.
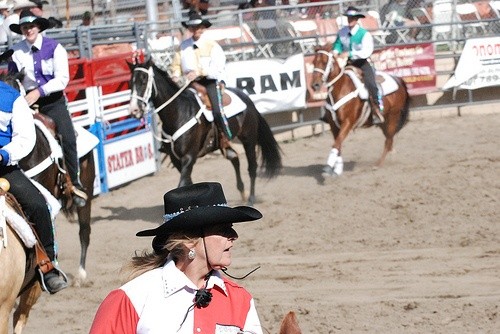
[66,0,500,64]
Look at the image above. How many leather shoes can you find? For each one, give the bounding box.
[43,272,68,293]
[225,149,237,158]
[372,114,381,124]
[72,193,86,207]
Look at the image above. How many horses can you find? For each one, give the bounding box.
[0,67,96,334]
[124,55,286,206]
[310,44,413,177]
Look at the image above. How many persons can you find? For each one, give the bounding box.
[169,6,239,160]
[90,182,264,334]
[2,0,95,42]
[0,76,69,294]
[331,5,385,127]
[1,7,89,207]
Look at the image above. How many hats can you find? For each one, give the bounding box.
[181,13,212,28]
[136,182,263,236]
[9,9,50,35]
[342,6,365,18]
